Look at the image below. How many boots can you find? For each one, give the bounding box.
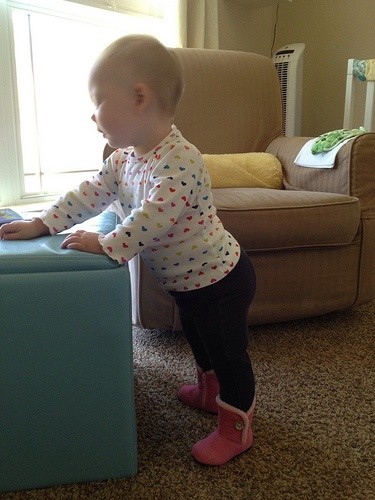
[177,364,219,412]
[191,393,257,466]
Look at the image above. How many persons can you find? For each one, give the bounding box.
[0,34,257,466]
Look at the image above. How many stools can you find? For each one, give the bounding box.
[0,210,138,492]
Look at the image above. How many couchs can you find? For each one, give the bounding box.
[100,47,375,333]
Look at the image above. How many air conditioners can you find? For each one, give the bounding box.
[274,42,305,137]
